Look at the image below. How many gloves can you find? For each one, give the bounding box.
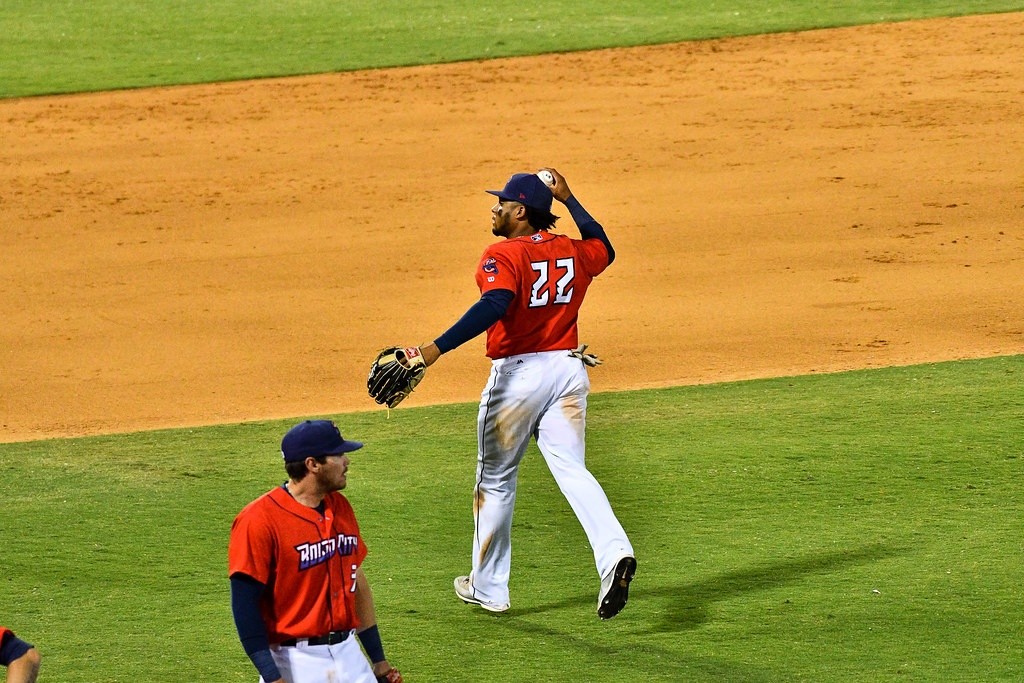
[571,344,602,368]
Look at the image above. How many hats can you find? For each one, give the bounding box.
[281,420,363,461]
[485,173,553,210]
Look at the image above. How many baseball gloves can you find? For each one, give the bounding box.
[366,345,428,410]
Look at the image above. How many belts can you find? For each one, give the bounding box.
[280,629,350,646]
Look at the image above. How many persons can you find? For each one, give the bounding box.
[364,169,637,621]
[226,421,402,683]
[0,625,41,683]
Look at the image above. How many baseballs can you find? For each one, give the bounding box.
[536,170,554,187]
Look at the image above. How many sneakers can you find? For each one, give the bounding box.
[454,576,511,612]
[597,554,637,620]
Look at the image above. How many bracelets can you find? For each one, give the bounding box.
[357,625,386,663]
